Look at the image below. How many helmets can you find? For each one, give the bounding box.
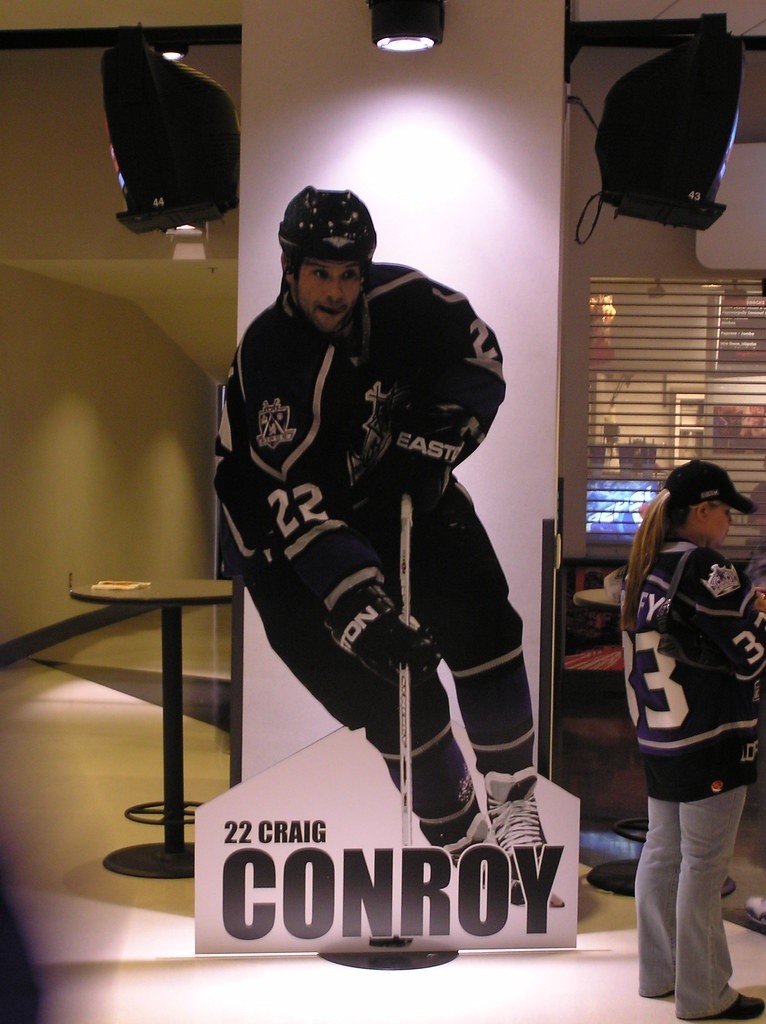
[278,186,377,262]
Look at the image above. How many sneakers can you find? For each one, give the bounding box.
[486,767,547,905]
[443,817,498,888]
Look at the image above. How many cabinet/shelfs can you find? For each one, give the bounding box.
[673,393,706,466]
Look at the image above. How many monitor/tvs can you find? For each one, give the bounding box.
[101,40,239,235]
[593,34,745,231]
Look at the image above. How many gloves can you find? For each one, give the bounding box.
[346,413,464,535]
[324,584,441,689]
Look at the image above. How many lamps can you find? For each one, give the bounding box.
[366,0,445,53]
[151,40,189,61]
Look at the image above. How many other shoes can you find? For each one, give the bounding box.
[744,896,766,923]
[678,994,765,1020]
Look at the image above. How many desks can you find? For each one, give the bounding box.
[68,577,234,881]
[571,587,738,902]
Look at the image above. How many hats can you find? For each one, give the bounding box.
[663,457,759,515]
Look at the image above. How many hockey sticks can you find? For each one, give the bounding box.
[387,495,417,847]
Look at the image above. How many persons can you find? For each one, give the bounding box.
[745,896,766,924]
[211,189,550,905]
[622,458,766,1021]
